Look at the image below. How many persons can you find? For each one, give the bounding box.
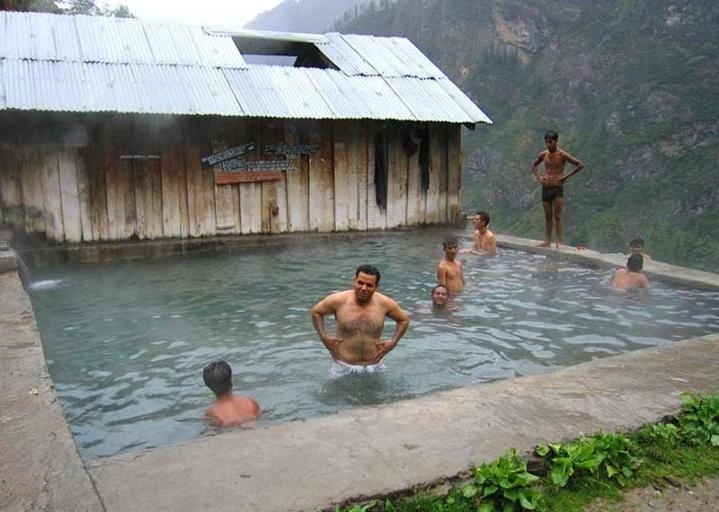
[609,253,650,292]
[526,252,562,321]
[627,237,652,259]
[457,211,496,256]
[530,130,585,250]
[437,235,467,299]
[415,281,464,316]
[202,360,259,428]
[310,263,413,378]
